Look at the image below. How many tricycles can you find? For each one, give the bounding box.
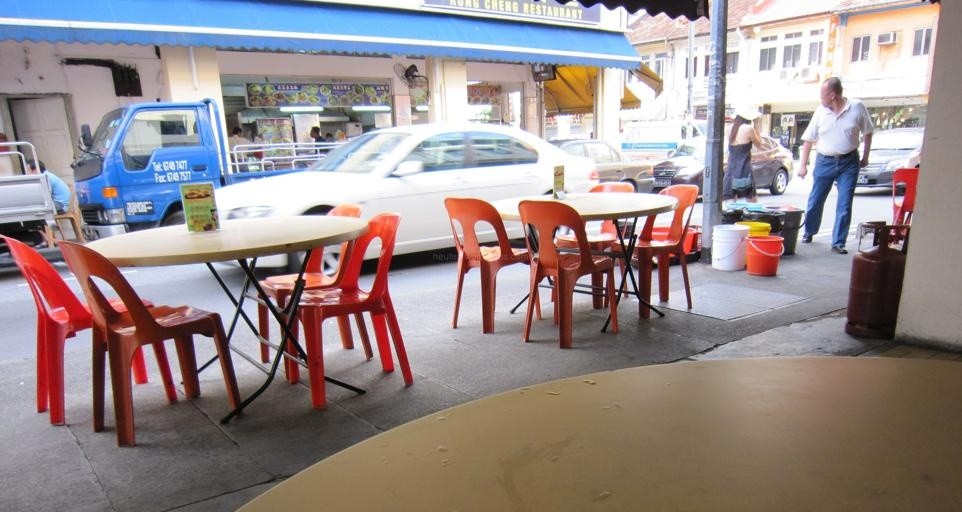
[0,141,57,238]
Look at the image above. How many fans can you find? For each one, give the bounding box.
[393,64,428,93]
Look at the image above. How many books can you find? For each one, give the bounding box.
[552,165,566,198]
[180,181,220,235]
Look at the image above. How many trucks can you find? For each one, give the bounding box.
[74,97,347,242]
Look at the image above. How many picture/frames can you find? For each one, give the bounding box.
[256,117,295,162]
[407,82,429,109]
[245,81,393,109]
[467,84,500,104]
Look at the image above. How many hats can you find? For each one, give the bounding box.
[735,101,763,121]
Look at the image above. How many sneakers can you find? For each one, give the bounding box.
[831,246,848,254]
[801,236,812,243]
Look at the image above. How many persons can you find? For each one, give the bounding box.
[310,127,326,155]
[230,129,260,174]
[723,96,763,204]
[324,134,334,141]
[24,157,71,249]
[797,77,874,254]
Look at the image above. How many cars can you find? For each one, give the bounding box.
[214,123,602,280]
[548,136,655,192]
[654,133,795,195]
[833,127,923,195]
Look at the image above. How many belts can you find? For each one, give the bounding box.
[824,152,852,159]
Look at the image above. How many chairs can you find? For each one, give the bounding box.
[628,182,700,321]
[0,232,149,425]
[42,183,85,249]
[518,200,621,350]
[557,181,636,308]
[443,197,542,335]
[257,204,373,381]
[54,238,244,448]
[284,213,417,413]
[892,167,919,227]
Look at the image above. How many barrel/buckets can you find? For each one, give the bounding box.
[712,224,749,271]
[747,235,785,276]
[733,222,772,236]
